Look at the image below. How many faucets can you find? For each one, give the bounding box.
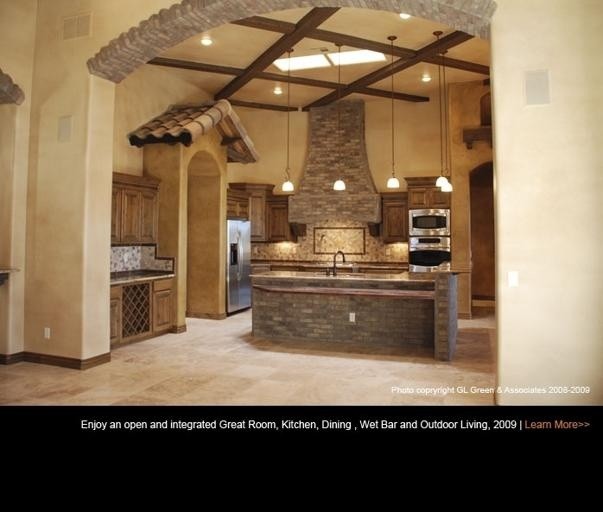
[332,251,346,275]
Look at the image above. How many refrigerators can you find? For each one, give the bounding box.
[226,219,251,314]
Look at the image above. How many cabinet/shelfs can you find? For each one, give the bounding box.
[228,196,248,218]
[407,187,450,208]
[248,192,265,241]
[109,286,121,343]
[267,202,287,240]
[153,279,173,331]
[383,200,407,241]
[112,182,156,244]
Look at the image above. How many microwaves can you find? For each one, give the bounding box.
[409,208,450,237]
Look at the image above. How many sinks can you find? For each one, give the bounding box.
[314,272,367,277]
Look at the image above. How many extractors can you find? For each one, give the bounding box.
[288,99,382,224]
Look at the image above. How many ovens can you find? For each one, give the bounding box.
[409,237,451,273]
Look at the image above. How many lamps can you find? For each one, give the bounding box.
[331,40,346,192]
[432,30,454,194]
[386,36,401,190]
[281,45,295,193]
